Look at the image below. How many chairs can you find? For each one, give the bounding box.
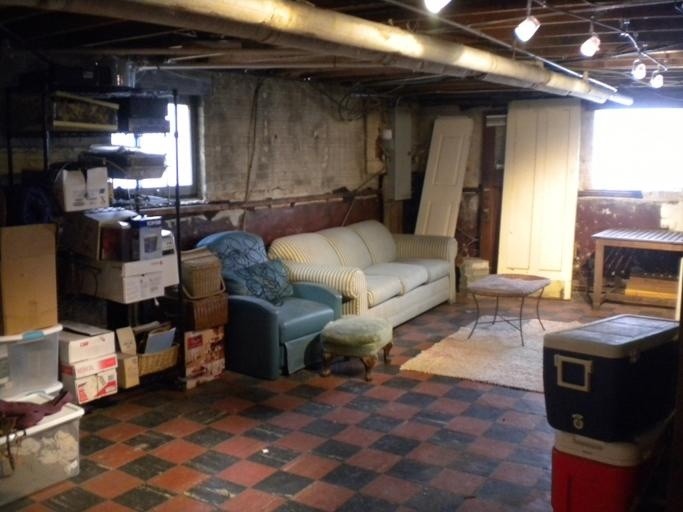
[198,231,342,381]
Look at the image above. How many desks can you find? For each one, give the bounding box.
[591,227,683,310]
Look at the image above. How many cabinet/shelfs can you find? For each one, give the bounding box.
[8,88,184,412]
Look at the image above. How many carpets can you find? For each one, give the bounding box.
[398,315,581,393]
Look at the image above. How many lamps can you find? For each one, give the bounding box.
[650,62,664,89]
[514,0,541,42]
[632,44,647,80]
[580,16,600,57]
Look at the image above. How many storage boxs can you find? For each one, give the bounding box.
[543,314,682,441]
[50,162,179,304]
[1,382,63,405]
[157,328,226,392]
[0,324,64,398]
[0,391,85,507]
[0,223,59,335]
[551,403,683,511]
[59,320,140,405]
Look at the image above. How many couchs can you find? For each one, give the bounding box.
[269,219,458,329]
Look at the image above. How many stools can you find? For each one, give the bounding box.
[319,315,395,382]
